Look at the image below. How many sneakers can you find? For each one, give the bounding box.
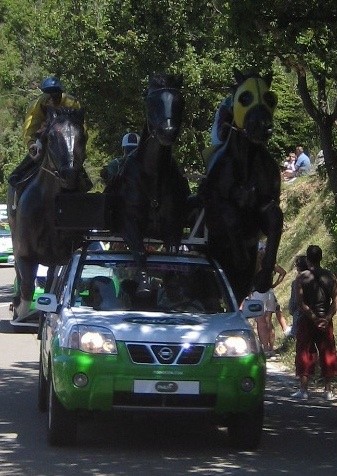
[325,389,336,401]
[291,390,308,400]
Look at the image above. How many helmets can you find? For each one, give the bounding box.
[41,76,63,89]
[122,133,141,147]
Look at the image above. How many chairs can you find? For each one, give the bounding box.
[89,276,116,297]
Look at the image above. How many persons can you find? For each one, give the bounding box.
[314,150,326,171]
[102,132,140,189]
[9,77,94,189]
[284,147,311,178]
[284,153,296,179]
[290,244,337,401]
[289,256,318,361]
[78,267,219,312]
[238,242,292,360]
[202,94,236,166]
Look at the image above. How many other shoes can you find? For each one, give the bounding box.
[263,326,292,358]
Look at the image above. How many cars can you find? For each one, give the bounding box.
[35,224,266,450]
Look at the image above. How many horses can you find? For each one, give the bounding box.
[9,105,103,322]
[104,72,198,261]
[198,67,284,309]
